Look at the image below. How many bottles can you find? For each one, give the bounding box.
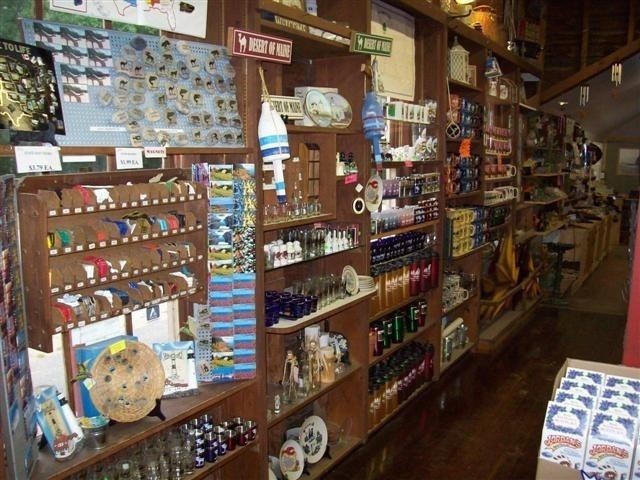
[262,100,439,431]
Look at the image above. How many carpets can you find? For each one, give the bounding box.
[329,305,629,480]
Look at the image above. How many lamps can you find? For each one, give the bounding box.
[610,56,623,87]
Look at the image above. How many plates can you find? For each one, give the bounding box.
[305,89,353,128]
[278,415,328,480]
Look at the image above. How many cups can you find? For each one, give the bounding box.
[65,414,262,480]
[442,93,520,364]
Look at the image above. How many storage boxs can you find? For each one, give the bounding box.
[535,357,640,480]
